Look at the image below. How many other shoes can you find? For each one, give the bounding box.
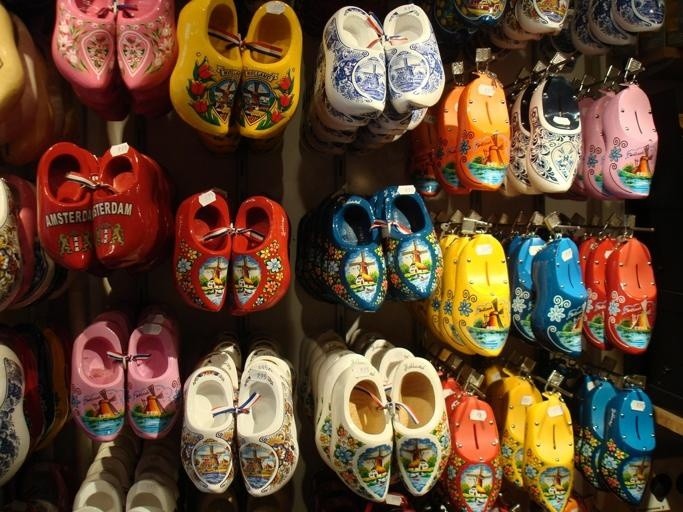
[1,0,672,512]
